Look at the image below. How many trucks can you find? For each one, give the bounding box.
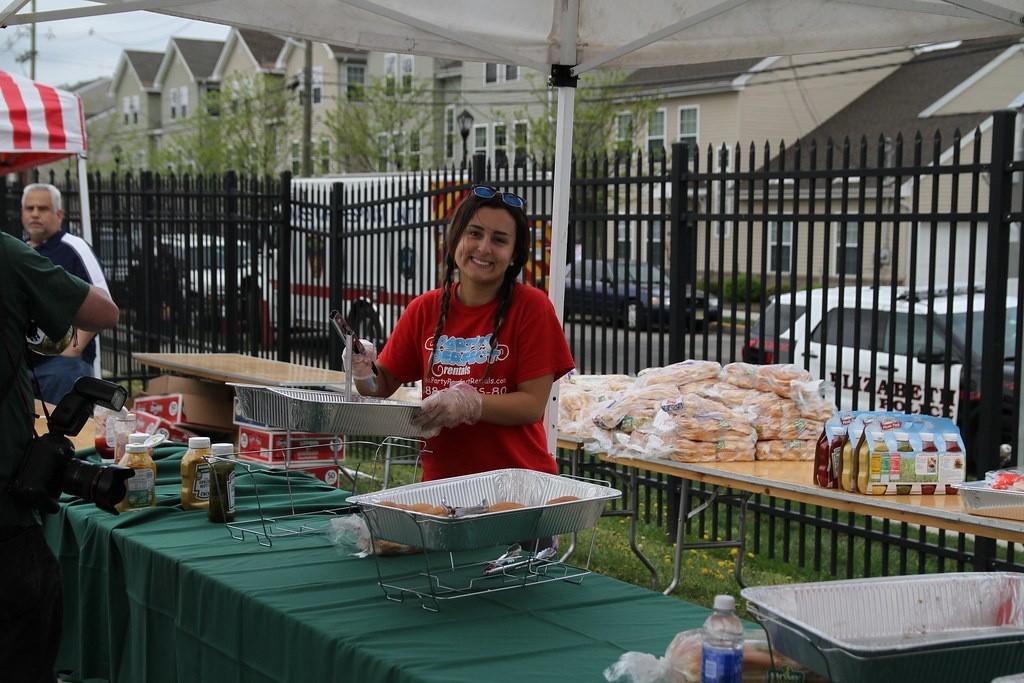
[240,169,554,377]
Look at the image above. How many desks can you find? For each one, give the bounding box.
[130,352,350,387]
[31,398,764,683]
[326,384,1024,595]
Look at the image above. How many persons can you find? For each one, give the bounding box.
[0,229,119,683]
[343,183,574,559]
[21,182,113,419]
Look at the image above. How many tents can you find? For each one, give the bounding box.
[0,68,101,382]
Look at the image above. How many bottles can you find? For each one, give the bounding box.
[818,427,962,495]
[208,443,236,524]
[701,594,742,683]
[181,437,210,510]
[94,405,157,511]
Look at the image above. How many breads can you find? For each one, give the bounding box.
[670,634,824,683]
[553,360,830,464]
[374,496,580,518]
[375,539,424,553]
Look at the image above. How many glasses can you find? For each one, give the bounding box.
[467,184,527,214]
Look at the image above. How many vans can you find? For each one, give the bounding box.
[128,232,250,335]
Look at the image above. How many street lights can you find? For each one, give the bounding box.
[456,110,473,170]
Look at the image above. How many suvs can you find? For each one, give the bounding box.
[741,283,1024,478]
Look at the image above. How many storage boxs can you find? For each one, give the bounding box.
[129,375,346,489]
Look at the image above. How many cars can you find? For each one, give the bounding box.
[531,259,723,338]
[97,229,136,287]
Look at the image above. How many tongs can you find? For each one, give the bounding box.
[330,309,379,402]
[477,543,556,579]
